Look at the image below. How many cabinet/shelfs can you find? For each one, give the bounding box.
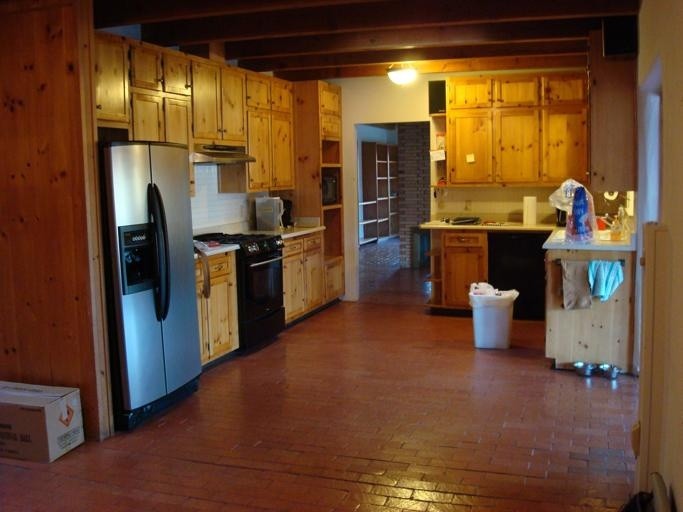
[361,140,400,239]
[544,231,636,374]
[295,81,353,301]
[90,30,129,129]
[192,245,241,366]
[544,68,593,189]
[421,220,488,315]
[247,226,324,328]
[129,39,195,197]
[218,71,295,194]
[449,71,543,188]
[191,54,248,145]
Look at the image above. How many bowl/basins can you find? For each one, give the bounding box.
[599,365,622,380]
[573,362,598,377]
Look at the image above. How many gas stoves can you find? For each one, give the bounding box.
[193,232,284,261]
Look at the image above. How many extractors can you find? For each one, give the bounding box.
[192,142,256,181]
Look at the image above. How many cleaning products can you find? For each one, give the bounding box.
[610,215,622,241]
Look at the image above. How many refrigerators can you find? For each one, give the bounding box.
[102,140,203,433]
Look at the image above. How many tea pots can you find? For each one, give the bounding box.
[255,197,284,231]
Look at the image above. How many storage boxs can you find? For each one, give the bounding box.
[0,381,85,464]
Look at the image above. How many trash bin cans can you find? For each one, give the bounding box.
[469,289,519,349]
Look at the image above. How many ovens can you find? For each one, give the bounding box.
[235,257,286,357]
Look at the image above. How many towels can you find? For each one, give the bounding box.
[560,260,593,310]
[588,260,624,303]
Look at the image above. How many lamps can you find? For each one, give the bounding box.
[386,68,417,85]
